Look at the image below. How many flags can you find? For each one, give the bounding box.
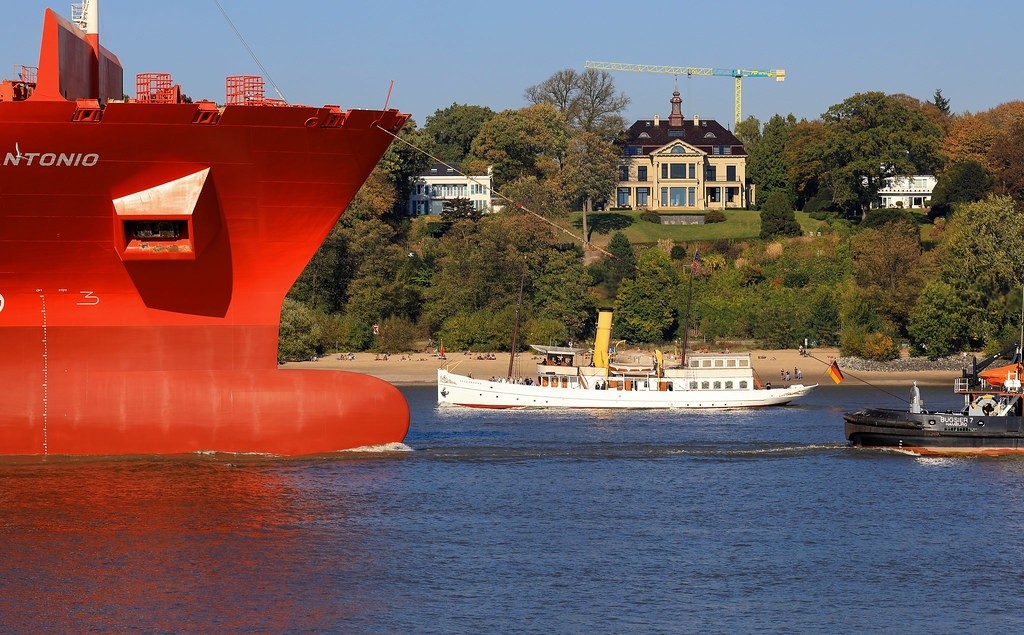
[441,343,444,356]
[827,360,844,385]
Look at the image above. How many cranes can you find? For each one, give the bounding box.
[584,58,786,142]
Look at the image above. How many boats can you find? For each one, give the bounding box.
[842,344,1024,463]
[436,246,823,413]
[609,363,656,376]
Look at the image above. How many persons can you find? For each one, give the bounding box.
[910,380,920,405]
[794,367,802,380]
[798,345,803,354]
[766,381,771,390]
[375,353,388,361]
[475,353,496,360]
[595,381,605,390]
[490,375,536,386]
[780,368,790,381]
[309,354,318,362]
[542,356,573,366]
[340,352,356,363]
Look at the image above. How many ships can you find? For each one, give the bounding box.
[0,0,414,458]
